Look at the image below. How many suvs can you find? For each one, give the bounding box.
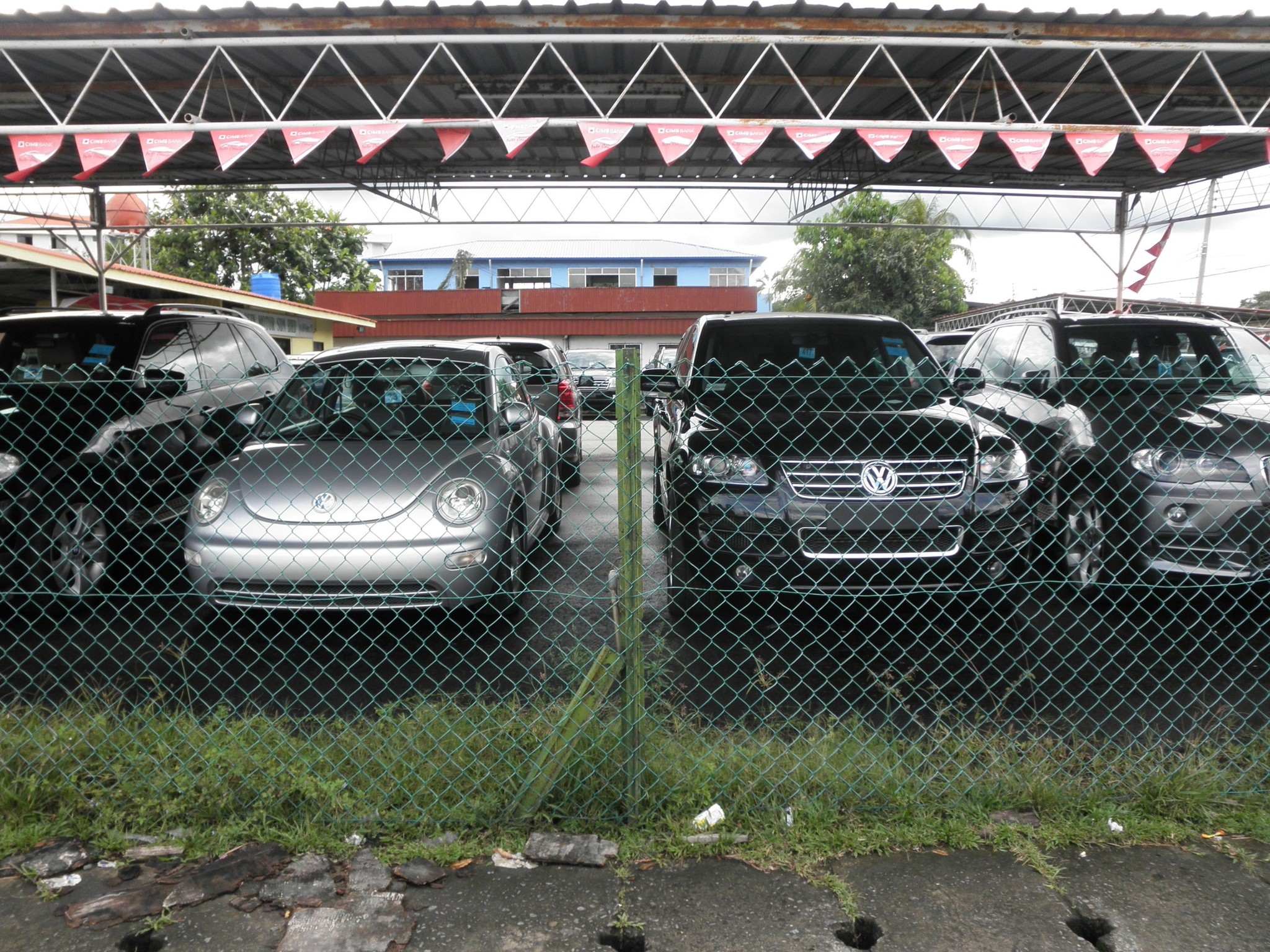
[950,312,1268,611]
[0,299,300,625]
[559,347,629,418]
[645,308,1035,630]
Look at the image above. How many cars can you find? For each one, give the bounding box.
[185,338,563,643]
[454,337,589,481]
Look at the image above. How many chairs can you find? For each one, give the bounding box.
[389,380,430,431]
[1130,333,1192,379]
[440,384,483,434]
[14,344,85,380]
[339,378,408,438]
[1090,351,1128,377]
[1067,344,1090,376]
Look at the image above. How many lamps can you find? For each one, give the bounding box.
[357,325,365,333]
